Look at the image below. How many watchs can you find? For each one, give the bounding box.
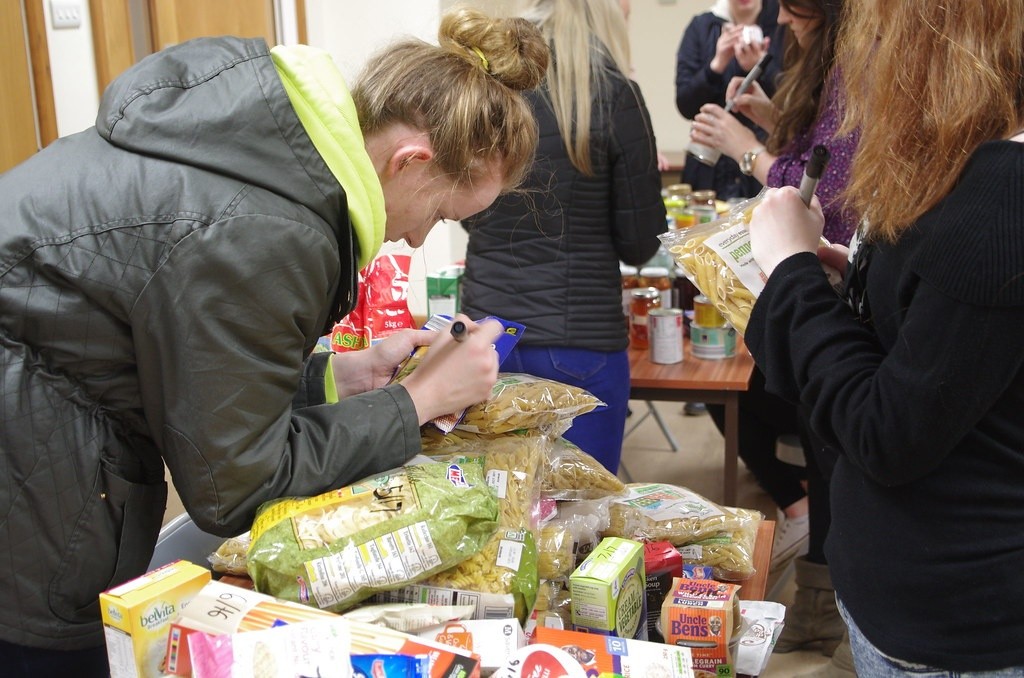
[739,143,768,178]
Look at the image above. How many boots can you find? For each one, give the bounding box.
[770,555,845,656]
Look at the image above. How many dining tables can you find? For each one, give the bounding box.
[621,336,758,507]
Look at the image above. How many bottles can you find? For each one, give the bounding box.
[612,185,739,370]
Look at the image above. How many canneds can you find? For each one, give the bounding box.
[620,266,685,364]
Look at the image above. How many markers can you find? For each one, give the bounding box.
[450,321,468,342]
[753,53,774,113]
[799,143,828,210]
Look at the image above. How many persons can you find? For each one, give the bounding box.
[742,0,1024,678]
[770,419,863,676]
[689,0,868,576]
[0,5,551,678]
[674,0,789,416]
[457,0,668,477]
